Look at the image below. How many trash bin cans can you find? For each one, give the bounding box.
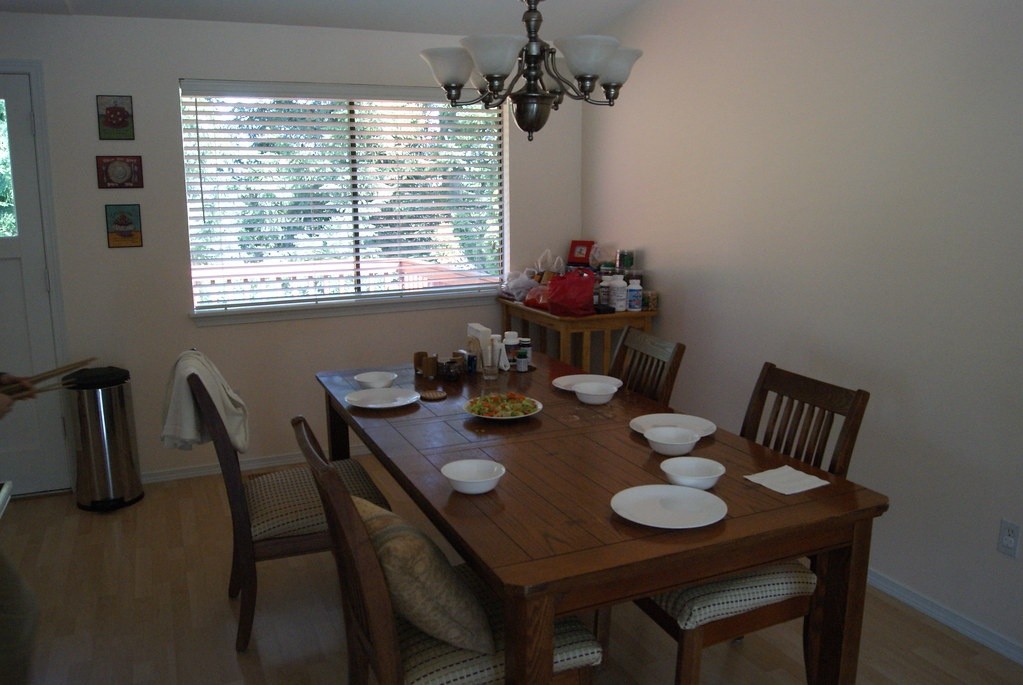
[61,367,144,512]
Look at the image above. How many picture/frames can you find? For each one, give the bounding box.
[96,155,143,189]
[105,204,143,248]
[96,94,135,140]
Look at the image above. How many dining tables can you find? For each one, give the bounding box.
[317,350,889,685]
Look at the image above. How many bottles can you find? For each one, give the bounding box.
[503,330,533,372]
[591,249,658,311]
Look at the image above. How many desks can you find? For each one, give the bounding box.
[495,295,658,375]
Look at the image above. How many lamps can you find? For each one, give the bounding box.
[420,0,644,140]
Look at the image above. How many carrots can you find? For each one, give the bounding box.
[481,391,525,416]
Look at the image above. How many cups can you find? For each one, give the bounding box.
[479,347,501,381]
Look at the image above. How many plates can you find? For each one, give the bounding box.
[629,413,717,437]
[345,387,421,408]
[463,396,543,419]
[552,374,623,391]
[611,484,728,528]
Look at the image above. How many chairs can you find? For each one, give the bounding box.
[607,327,687,406]
[289,415,603,685]
[186,349,393,651]
[595,360,871,685]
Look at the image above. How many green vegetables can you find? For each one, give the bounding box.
[467,395,537,416]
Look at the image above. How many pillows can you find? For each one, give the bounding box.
[351,496,496,656]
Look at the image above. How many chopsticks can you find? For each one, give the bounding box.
[0,357,98,400]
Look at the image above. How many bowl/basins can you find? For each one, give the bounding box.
[659,456,726,490]
[440,459,505,494]
[354,371,398,389]
[644,426,700,455]
[572,382,617,405]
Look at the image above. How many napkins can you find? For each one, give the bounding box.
[743,464,831,493]
